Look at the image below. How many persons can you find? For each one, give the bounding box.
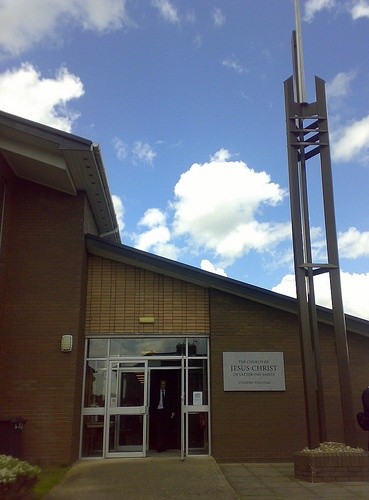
[149,379,176,453]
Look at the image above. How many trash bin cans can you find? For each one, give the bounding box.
[0,415,29,460]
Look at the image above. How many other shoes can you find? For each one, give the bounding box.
[157,448,166,453]
